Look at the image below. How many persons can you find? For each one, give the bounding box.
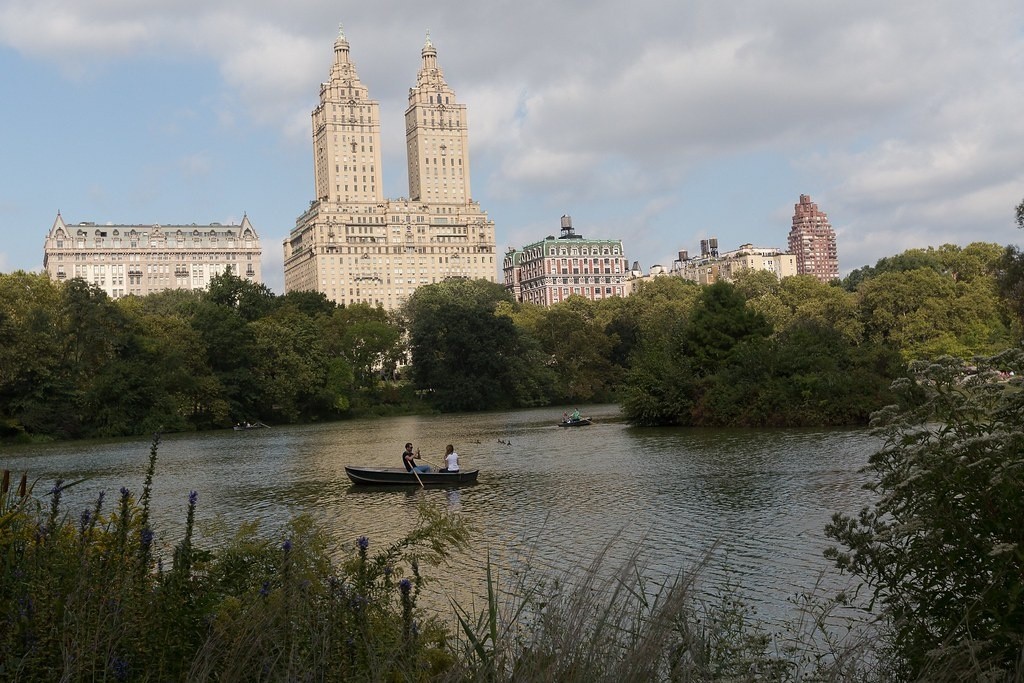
[402,443,431,473]
[439,444,460,473]
[246,422,250,427]
[562,408,580,423]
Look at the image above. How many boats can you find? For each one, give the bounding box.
[557,417,593,427]
[233,422,262,430]
[346,465,481,487]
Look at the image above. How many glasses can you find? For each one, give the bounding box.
[410,447,413,450]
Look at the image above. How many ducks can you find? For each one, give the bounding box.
[497,437,512,447]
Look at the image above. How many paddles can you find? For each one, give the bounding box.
[420,458,445,469]
[407,460,425,488]
